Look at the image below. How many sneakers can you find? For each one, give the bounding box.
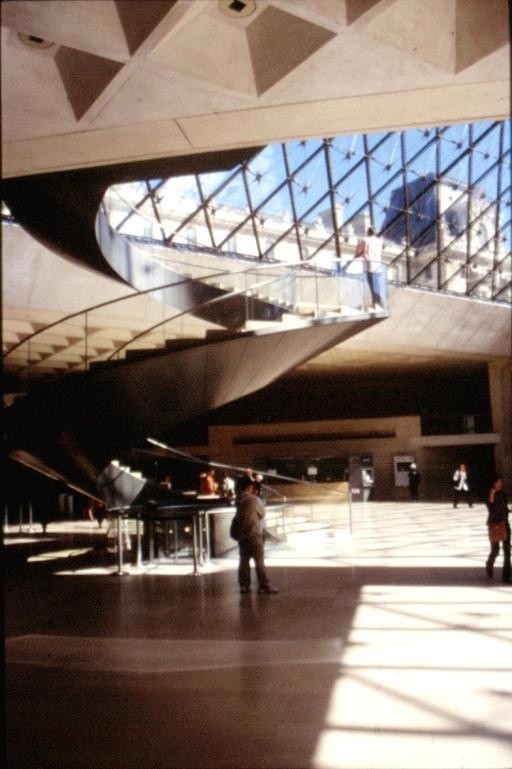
[259,587,279,593]
[241,586,252,592]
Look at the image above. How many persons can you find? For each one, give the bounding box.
[198,466,280,596]
[161,476,173,490]
[409,462,420,499]
[487,477,512,584]
[357,224,384,312]
[452,462,473,508]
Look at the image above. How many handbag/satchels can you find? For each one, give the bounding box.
[231,517,239,540]
[488,521,507,543]
